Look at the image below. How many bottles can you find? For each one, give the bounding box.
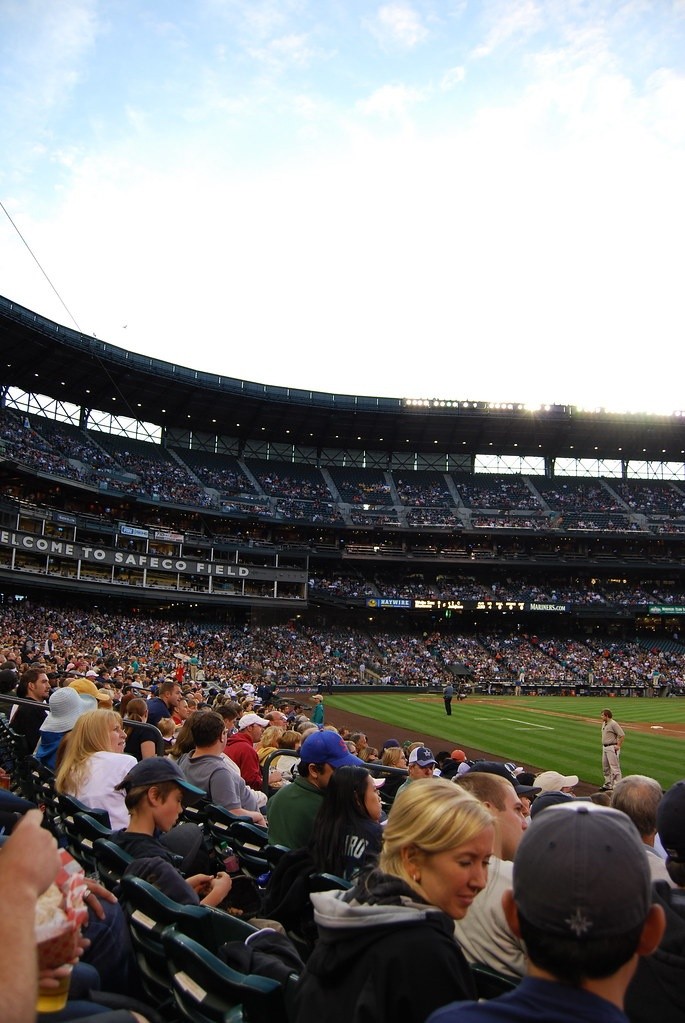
[220,841,239,872]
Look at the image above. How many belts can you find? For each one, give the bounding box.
[604,743,617,747]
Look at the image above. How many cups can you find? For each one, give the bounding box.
[35,920,77,1012]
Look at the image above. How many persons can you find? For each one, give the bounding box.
[0,407,685,1023]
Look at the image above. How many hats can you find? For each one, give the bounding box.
[533,771,579,796]
[403,739,412,747]
[450,750,465,762]
[511,802,655,938]
[383,739,399,751]
[409,747,437,767]
[504,762,523,772]
[117,757,206,803]
[312,694,323,701]
[298,730,365,769]
[656,781,685,864]
[530,790,592,819]
[238,713,270,731]
[86,670,99,677]
[68,678,110,701]
[467,761,542,795]
[39,686,97,732]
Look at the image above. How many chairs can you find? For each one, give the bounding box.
[0,712,519,1023]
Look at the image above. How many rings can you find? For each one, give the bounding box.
[83,889,91,899]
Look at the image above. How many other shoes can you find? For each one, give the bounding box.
[597,786,613,791]
